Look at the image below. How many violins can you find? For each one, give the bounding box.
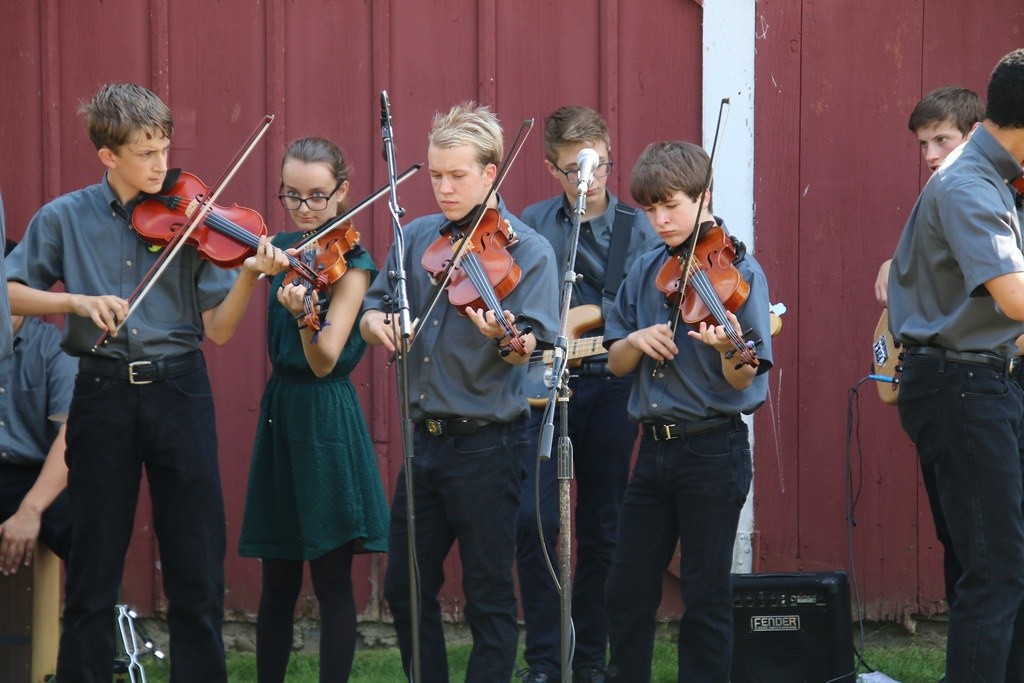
[653,225,765,372]
[131,171,332,293]
[419,206,534,360]
[281,216,361,334]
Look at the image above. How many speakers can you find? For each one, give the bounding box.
[726,569,856,683]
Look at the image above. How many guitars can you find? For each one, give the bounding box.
[522,301,789,410]
[871,162,1024,405]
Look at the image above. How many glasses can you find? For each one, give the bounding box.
[549,151,613,183]
[278,179,343,211]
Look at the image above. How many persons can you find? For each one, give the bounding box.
[602,140,773,683]
[359,101,563,683]
[236,137,391,683]
[5,81,296,683]
[875,86,987,606]
[886,49,1024,683]
[0,195,78,634]
[516,104,665,683]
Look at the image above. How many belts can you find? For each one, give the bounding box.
[573,362,613,375]
[903,344,1024,375]
[420,418,504,436]
[79,351,204,384]
[645,415,742,441]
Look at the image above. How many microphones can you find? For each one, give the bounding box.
[379,90,398,162]
[577,147,599,196]
